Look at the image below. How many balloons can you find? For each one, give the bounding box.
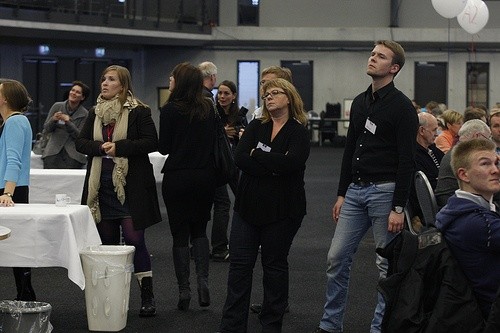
[457,0,489,34]
[431,0,467,18]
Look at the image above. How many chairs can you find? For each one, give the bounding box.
[316,111,338,144]
[414,169,440,229]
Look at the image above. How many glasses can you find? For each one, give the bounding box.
[262,90,288,100]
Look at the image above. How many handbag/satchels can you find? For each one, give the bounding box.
[209,99,235,186]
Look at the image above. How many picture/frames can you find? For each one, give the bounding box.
[157,85,172,109]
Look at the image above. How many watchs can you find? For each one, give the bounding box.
[3,193,13,198]
[391,205,404,214]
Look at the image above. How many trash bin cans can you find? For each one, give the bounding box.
[80,245,136,332]
[0,301,52,333]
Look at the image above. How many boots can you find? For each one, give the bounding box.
[173,246,191,312]
[192,237,210,306]
[136,276,155,315]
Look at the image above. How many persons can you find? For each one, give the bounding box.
[315,40,420,333]
[0,78,36,302]
[75,64,161,316]
[408,99,500,333]
[39,81,90,169]
[158,62,311,333]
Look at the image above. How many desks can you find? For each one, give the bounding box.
[28,168,88,204]
[308,117,352,147]
[0,198,102,290]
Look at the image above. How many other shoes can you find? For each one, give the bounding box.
[213,251,230,262]
[249,302,289,313]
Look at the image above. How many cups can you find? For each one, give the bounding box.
[55,194,71,207]
